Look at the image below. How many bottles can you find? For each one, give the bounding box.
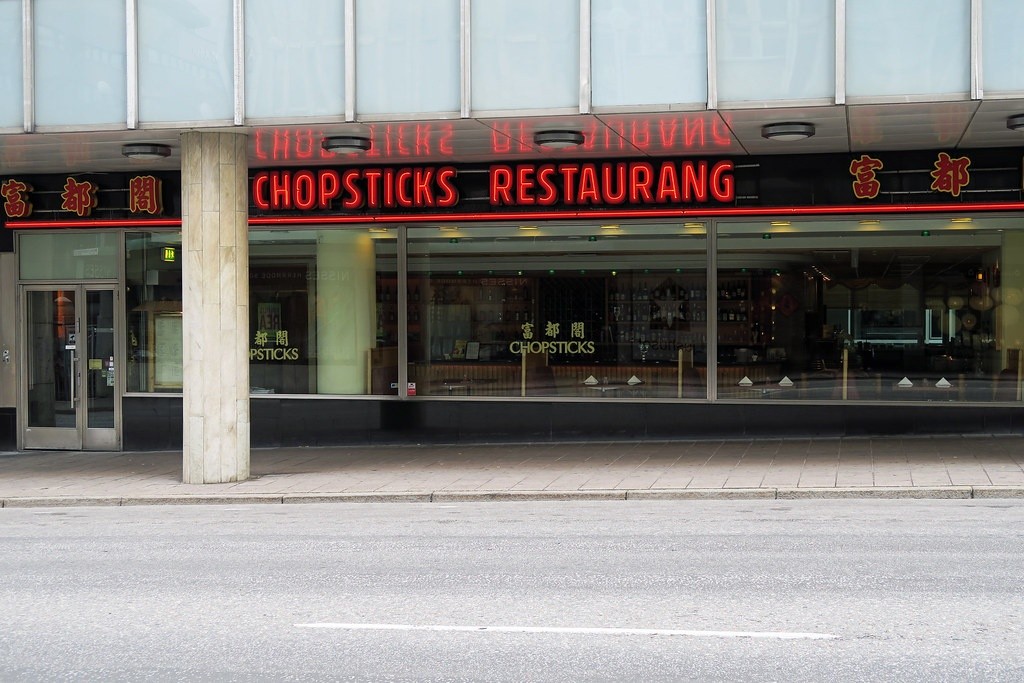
[609,278,752,367]
[373,281,536,366]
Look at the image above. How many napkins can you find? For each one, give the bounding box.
[738,376,753,387]
[935,376,953,389]
[627,375,642,385]
[584,375,599,385]
[778,376,794,386]
[897,377,914,388]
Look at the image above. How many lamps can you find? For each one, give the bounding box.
[1007,117,1024,132]
[761,125,816,141]
[122,145,171,161]
[534,133,585,149]
[321,139,372,154]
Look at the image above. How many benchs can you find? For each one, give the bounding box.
[991,369,1019,401]
[833,371,867,398]
[674,348,706,398]
[511,349,559,396]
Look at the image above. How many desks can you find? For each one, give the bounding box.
[737,381,794,392]
[585,381,644,397]
[892,378,960,401]
[429,378,499,396]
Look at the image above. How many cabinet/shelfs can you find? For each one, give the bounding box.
[472,299,532,344]
[605,275,750,354]
[373,303,424,361]
